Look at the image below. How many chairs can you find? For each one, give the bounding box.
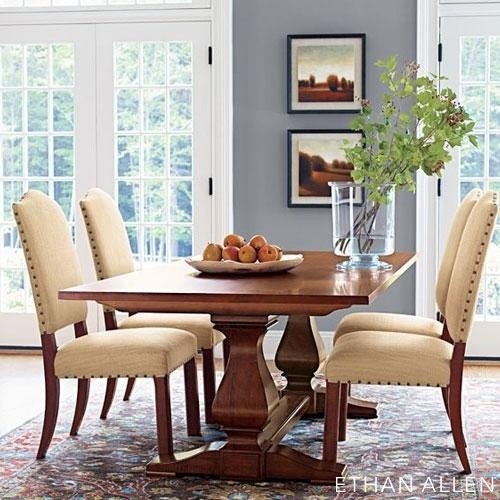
[11,186,204,463]
[78,185,228,426]
[310,190,500,477]
[326,188,485,443]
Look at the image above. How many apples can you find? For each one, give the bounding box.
[202,233,284,264]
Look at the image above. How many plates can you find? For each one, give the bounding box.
[183,253,303,275]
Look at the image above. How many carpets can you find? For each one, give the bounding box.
[1,371,498,500]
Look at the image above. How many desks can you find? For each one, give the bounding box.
[55,244,425,486]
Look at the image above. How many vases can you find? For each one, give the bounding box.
[327,181,400,275]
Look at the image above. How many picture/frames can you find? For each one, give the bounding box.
[285,31,368,115]
[285,126,370,210]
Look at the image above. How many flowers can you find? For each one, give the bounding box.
[334,54,480,255]
